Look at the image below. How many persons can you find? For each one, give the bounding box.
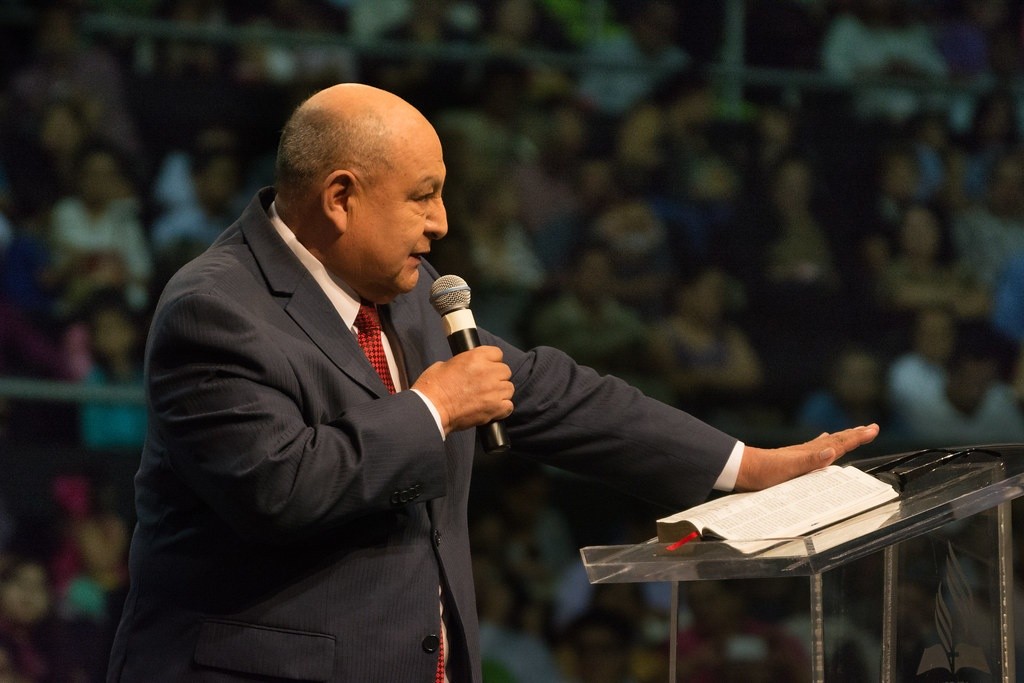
[1,1,1023,683]
[107,81,880,683]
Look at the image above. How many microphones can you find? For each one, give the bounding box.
[429,275,511,454]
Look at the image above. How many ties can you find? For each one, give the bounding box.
[354,305,446,683]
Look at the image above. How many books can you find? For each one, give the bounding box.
[657,465,900,555]
